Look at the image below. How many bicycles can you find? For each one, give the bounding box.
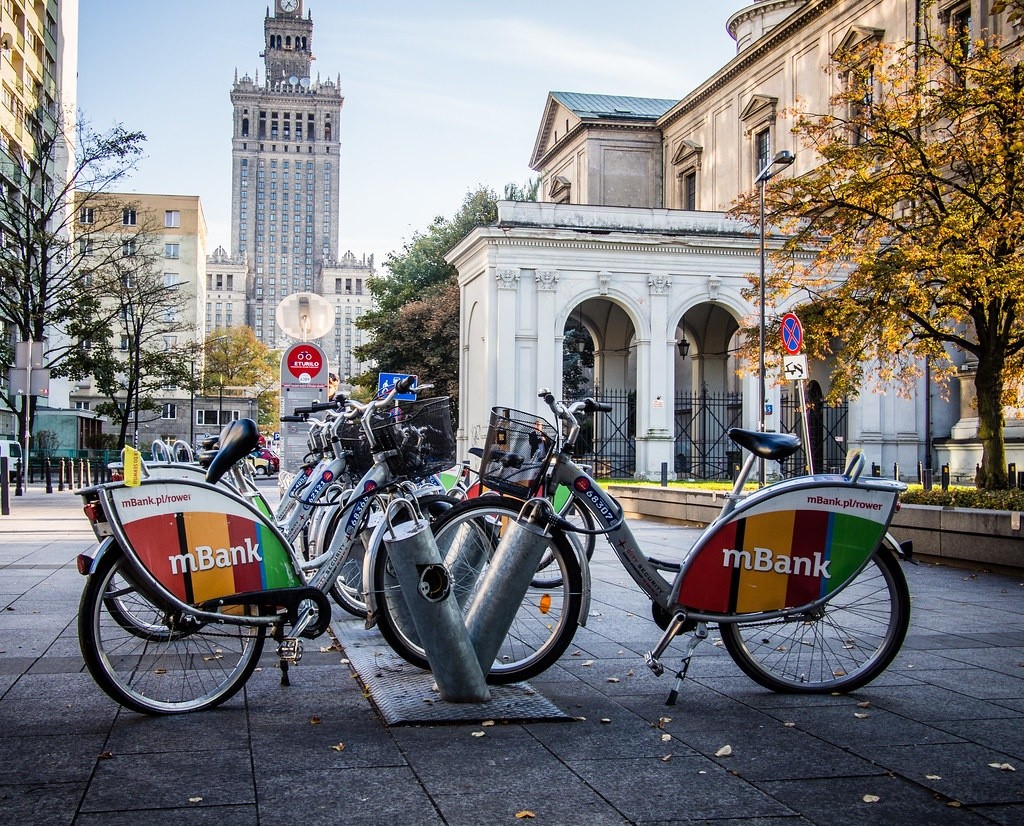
[66,356,915,722]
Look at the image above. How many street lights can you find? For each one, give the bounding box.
[756,148,795,489]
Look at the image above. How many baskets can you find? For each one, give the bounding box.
[308,396,459,481]
[479,406,559,498]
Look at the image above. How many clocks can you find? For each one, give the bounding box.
[278,0,299,13]
